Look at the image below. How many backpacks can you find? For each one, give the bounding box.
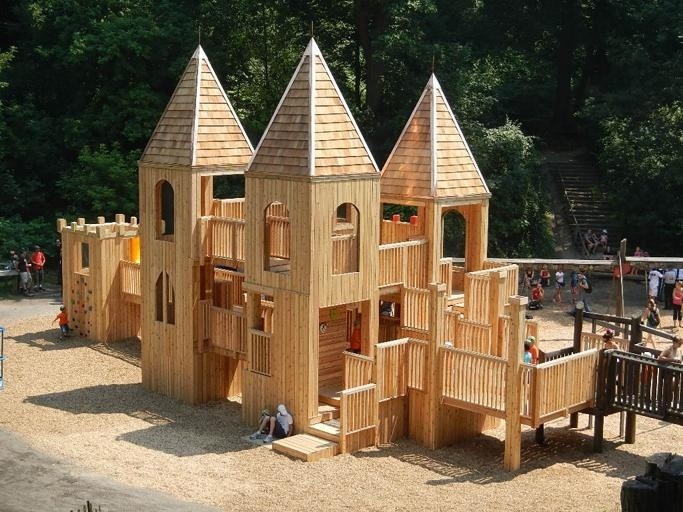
[648,307,660,327]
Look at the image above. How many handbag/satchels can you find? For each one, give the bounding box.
[582,278,593,293]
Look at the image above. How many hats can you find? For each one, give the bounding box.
[278,404,288,416]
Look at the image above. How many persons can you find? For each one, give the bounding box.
[4,239,62,297]
[51,303,71,340]
[657,335,683,417]
[637,351,654,395]
[379,301,397,315]
[642,299,663,350]
[522,229,682,332]
[598,327,622,367]
[523,340,531,365]
[526,335,539,365]
[249,403,295,445]
[348,320,364,354]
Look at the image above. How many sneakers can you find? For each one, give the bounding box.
[250,433,262,440]
[264,436,273,442]
